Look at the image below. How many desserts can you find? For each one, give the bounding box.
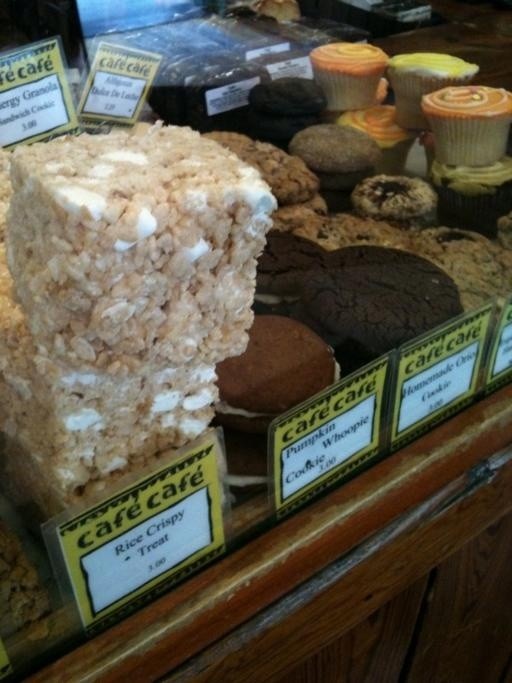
[0,1,512,641]
